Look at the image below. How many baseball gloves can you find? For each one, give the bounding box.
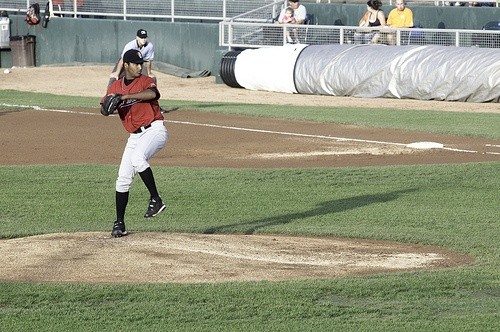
[100,93,123,116]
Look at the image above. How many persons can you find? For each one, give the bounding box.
[354,0,414,45]
[100,28,169,235]
[278,0,306,46]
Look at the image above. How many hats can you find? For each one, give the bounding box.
[137,29,149,38]
[124,49,148,63]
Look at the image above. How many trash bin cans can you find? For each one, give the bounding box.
[9,34,36,67]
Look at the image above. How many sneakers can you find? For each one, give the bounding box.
[144,197,166,218]
[112,220,127,237]
